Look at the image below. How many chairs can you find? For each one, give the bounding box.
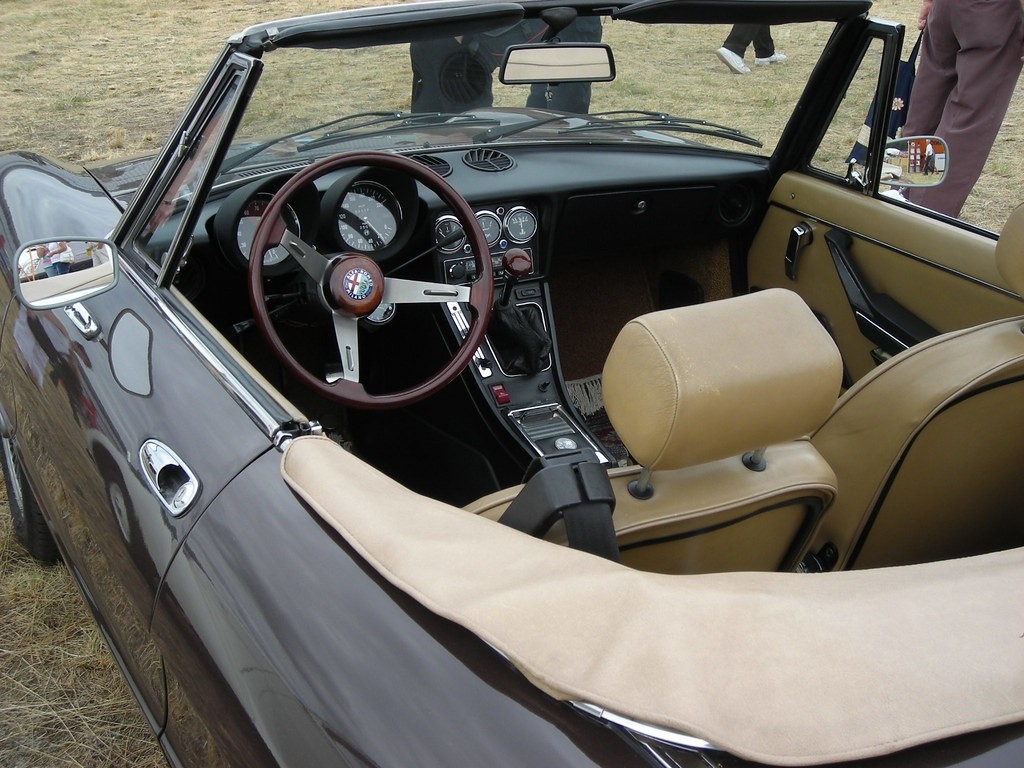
[462,287,844,576]
[800,201,1024,574]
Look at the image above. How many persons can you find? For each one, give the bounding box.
[85,241,109,267]
[890,0,1024,220]
[716,23,786,74]
[923,139,935,175]
[26,307,92,393]
[410,35,493,114]
[37,241,74,277]
[146,88,235,233]
[526,16,603,113]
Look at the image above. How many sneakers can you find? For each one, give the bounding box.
[755,53,786,67]
[716,47,751,74]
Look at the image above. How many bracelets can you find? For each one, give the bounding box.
[162,199,172,206]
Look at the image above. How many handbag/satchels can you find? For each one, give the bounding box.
[845,25,925,167]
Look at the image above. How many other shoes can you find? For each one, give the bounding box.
[931,171,934,175]
[923,172,928,175]
[936,168,938,173]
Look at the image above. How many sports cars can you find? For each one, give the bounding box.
[0,0,1024,768]
